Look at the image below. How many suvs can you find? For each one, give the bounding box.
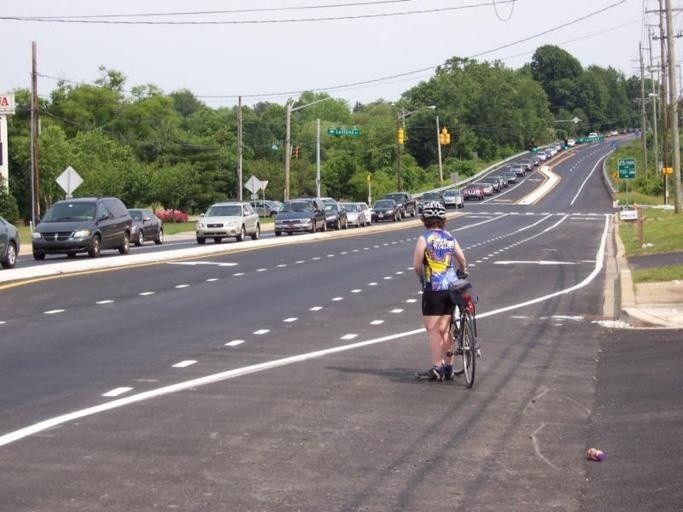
[442,189,463,209]
[484,177,500,193]
[518,159,533,170]
[510,164,526,177]
[463,184,484,200]
[418,193,445,214]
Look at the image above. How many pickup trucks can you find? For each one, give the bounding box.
[384,192,416,218]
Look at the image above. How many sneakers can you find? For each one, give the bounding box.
[415,364,454,382]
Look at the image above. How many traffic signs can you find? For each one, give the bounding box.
[327,129,359,136]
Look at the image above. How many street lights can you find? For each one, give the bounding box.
[284,94,346,201]
[398,104,437,192]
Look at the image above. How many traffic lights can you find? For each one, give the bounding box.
[289,145,296,160]
[528,146,536,151]
[395,128,402,145]
[439,129,450,145]
[367,175,372,181]
[296,144,301,161]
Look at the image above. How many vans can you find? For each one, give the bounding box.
[31,197,131,257]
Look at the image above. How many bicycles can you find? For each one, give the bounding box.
[418,271,481,388]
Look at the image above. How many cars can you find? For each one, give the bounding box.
[482,182,493,195]
[319,197,336,206]
[194,201,261,244]
[344,203,364,227]
[535,152,547,161]
[273,200,326,237]
[0,216,20,268]
[495,175,508,188]
[369,199,401,223]
[126,208,163,247]
[250,200,280,218]
[545,127,641,159]
[353,202,371,226]
[504,172,517,183]
[530,157,539,165]
[272,200,282,206]
[324,204,348,231]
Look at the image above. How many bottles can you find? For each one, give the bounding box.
[585,446,604,462]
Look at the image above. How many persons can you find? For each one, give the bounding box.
[413,200,466,382]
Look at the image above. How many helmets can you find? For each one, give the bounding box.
[422,200,446,220]
